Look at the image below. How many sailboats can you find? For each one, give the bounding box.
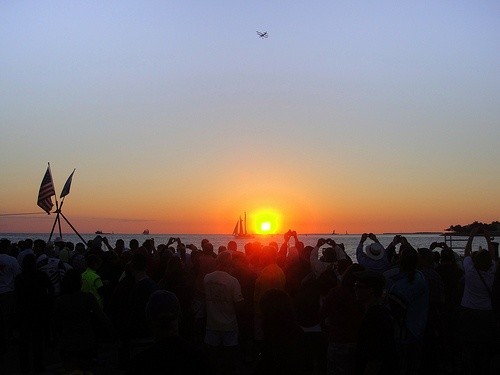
[232,212,255,240]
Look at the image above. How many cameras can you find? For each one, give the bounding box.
[186,244,190,248]
[288,232,296,235]
[366,234,370,237]
[396,235,401,237]
[174,238,179,241]
[323,240,328,243]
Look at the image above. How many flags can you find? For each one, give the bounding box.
[36,167,55,215]
[59,171,73,198]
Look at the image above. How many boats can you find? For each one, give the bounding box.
[94,230,103,234]
[142,229,150,235]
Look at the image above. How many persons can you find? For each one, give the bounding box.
[463,227,498,331]
[202,251,245,350]
[0,231,500,353]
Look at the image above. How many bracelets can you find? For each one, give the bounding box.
[468,236,473,241]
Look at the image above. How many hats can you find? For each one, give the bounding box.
[472,249,492,272]
[53,237,63,242]
[366,243,385,260]
[47,241,54,247]
